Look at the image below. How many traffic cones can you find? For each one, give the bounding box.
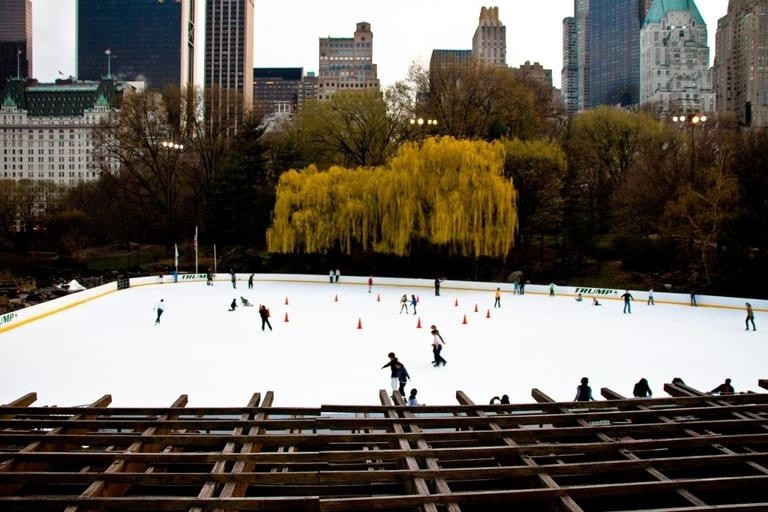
[356,317,363,329]
[474,304,479,312]
[376,293,381,302]
[454,297,459,306]
[416,295,420,302]
[334,293,339,302]
[256,296,291,324]
[415,316,422,328]
[461,314,468,324]
[486,309,491,318]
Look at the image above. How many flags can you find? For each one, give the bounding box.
[174,244,180,267]
[194,231,197,253]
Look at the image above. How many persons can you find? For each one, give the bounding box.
[206,263,216,286]
[690,288,697,306]
[239,296,255,307]
[408,294,417,315]
[591,296,601,306]
[259,305,272,332]
[229,267,236,289]
[632,377,653,400]
[435,275,444,296]
[549,282,554,296]
[227,298,239,311]
[152,298,164,326]
[392,361,411,403]
[380,351,400,398]
[430,329,447,367]
[517,278,529,295]
[744,302,756,331]
[512,277,519,295]
[488,394,513,414]
[574,293,583,301]
[328,267,335,283]
[647,286,656,305]
[572,376,596,401]
[248,272,255,289]
[335,268,341,282]
[367,275,374,294]
[399,294,411,314]
[430,325,447,364]
[403,388,426,406]
[706,378,734,397]
[157,268,165,285]
[493,287,501,308]
[619,288,636,314]
[173,270,177,283]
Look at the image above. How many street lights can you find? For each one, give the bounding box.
[407,116,439,145]
[670,112,708,291]
[105,47,114,80]
[161,139,184,261]
[15,47,24,79]
